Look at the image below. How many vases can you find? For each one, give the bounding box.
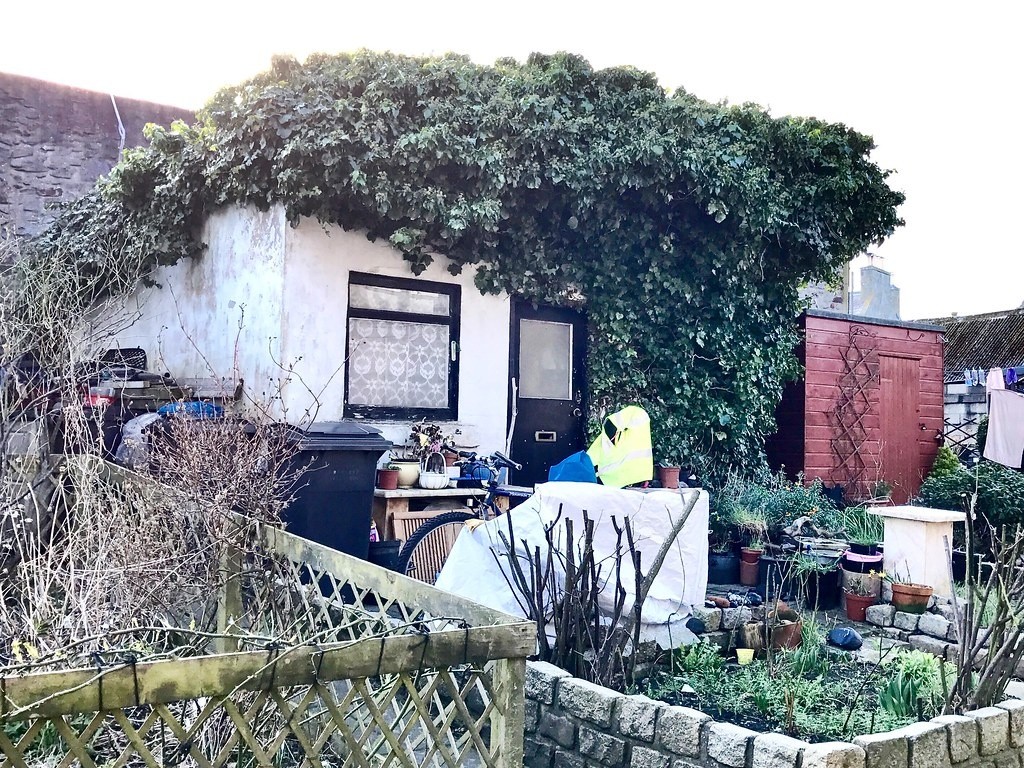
[388,458,421,488]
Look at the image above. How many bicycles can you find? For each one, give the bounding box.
[394,450,535,631]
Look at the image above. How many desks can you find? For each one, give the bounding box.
[373,487,510,543]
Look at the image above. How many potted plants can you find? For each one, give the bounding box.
[709,473,885,619]
[378,462,400,489]
[659,458,681,489]
[891,583,933,613]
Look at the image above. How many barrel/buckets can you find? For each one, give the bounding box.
[735,649,755,665]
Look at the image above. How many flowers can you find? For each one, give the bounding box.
[867,558,912,585]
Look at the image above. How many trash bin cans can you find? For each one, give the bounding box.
[142,417,256,496]
[271,422,394,604]
